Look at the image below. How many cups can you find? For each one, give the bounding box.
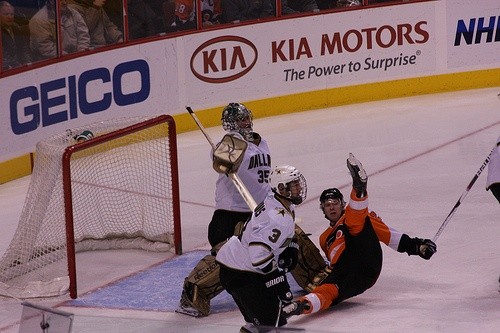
[76,131,93,141]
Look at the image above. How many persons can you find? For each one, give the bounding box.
[175,102,327,318]
[214,164,308,332]
[0,0,373,71]
[281,152,437,317]
[485,138,500,292]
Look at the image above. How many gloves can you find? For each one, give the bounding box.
[278,238,299,272]
[261,268,293,307]
[409,237,437,260]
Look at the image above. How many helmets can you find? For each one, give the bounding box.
[320,188,344,203]
[221,103,252,131]
[268,165,301,194]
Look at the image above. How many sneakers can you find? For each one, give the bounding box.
[281,300,302,317]
[346,153,368,192]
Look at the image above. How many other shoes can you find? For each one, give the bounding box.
[238,321,277,332]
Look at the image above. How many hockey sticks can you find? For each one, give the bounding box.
[185,105,259,214]
[421,137,500,257]
